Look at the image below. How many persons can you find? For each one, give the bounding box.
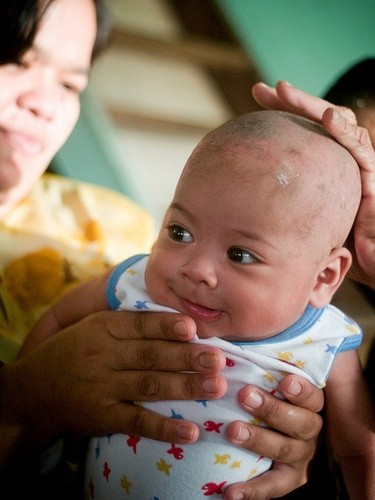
[0,108,375,499]
[247,55,375,437]
[0,0,326,499]
